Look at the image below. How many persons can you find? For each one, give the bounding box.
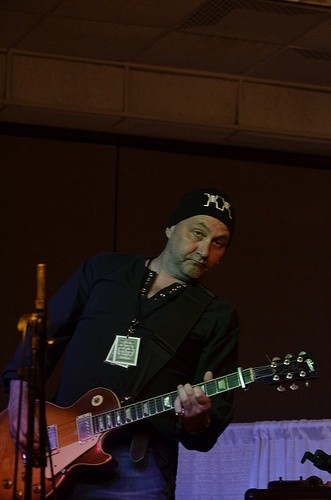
[5,187,241,500]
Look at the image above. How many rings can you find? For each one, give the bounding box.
[174,407,185,418]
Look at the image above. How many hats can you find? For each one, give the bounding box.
[168,187,241,234]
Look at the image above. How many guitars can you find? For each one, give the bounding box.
[0,351,316,500]
